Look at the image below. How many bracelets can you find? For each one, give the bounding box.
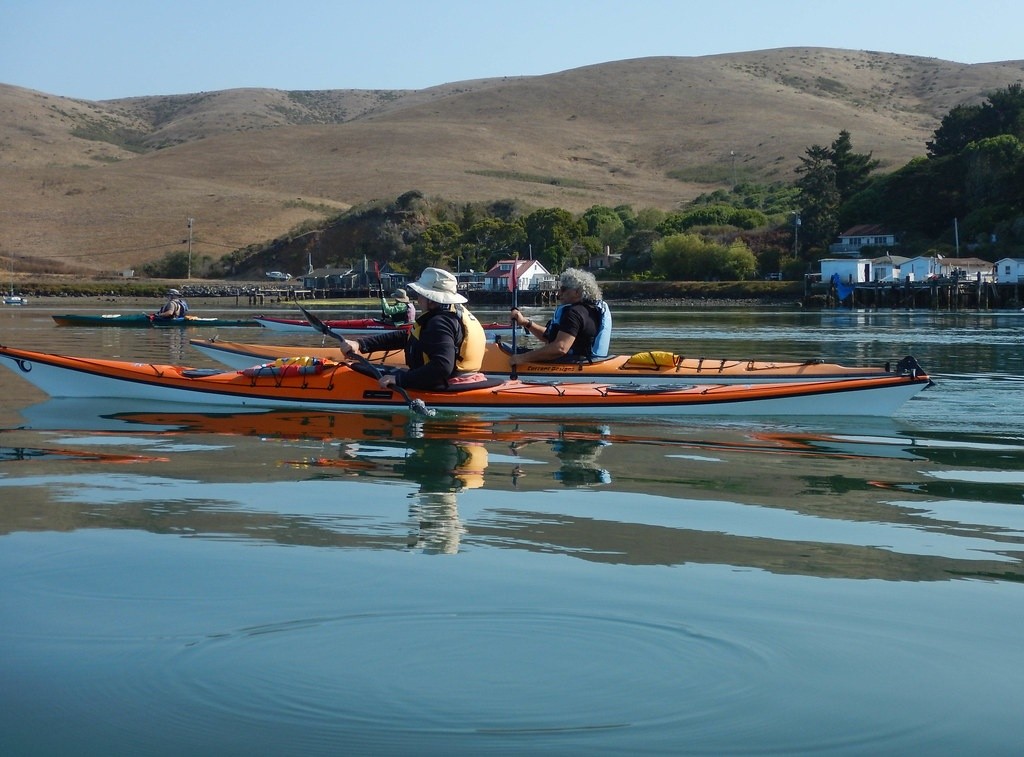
[525,318,533,328]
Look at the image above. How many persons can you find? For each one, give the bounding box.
[158,289,182,317]
[378,288,410,326]
[341,267,468,389]
[508,267,602,366]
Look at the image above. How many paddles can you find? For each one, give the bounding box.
[290,292,432,419]
[373,258,386,318]
[506,249,522,378]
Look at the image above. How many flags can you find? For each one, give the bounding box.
[797,216,802,225]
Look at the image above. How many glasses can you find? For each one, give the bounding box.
[560,286,575,292]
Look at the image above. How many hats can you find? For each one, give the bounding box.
[407,267,469,304]
[391,289,409,302]
[166,288,182,296]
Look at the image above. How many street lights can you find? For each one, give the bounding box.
[794,211,801,260]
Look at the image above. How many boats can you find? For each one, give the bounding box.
[50,313,261,327]
[253,315,526,336]
[0,344,937,423]
[2,295,28,307]
[186,335,898,376]
